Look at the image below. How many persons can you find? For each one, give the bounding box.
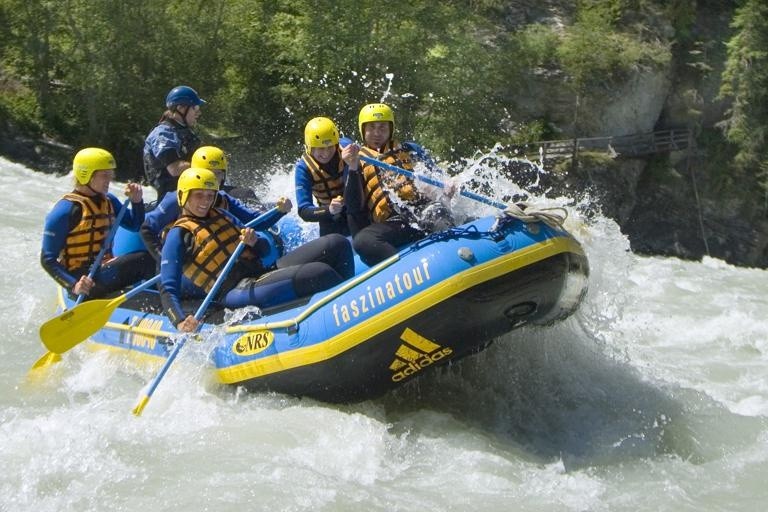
[144,85,266,211]
[140,146,293,303]
[296,117,358,236]
[158,165,354,332]
[39,146,161,307]
[342,104,457,268]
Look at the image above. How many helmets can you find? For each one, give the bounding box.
[177,166,219,209]
[304,117,339,156]
[358,103,395,142]
[191,145,227,180]
[72,147,117,187]
[166,86,207,108]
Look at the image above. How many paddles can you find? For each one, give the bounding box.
[30,183,137,369]
[131,232,245,416]
[39,197,288,354]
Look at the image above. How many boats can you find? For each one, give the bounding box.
[56,210,590,404]
